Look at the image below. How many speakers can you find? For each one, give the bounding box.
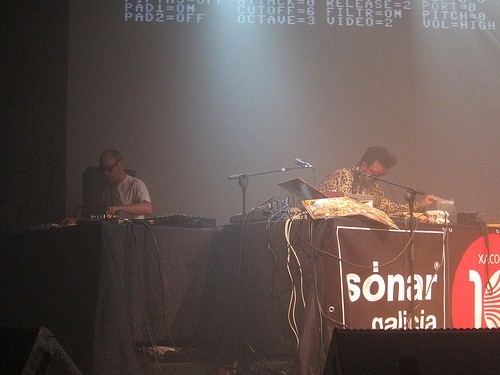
[0,326,82,375]
[322,326,500,375]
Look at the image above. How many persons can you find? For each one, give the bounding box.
[316,145,441,215]
[60,149,152,224]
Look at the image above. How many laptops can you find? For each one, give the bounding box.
[278,178,329,200]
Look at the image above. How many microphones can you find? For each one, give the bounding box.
[295,158,312,168]
[352,166,359,194]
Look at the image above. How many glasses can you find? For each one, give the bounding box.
[100,161,119,172]
